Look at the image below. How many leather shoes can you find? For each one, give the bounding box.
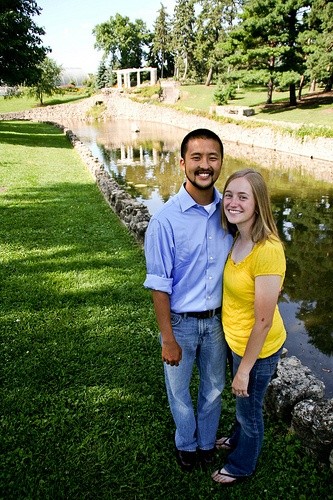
[197,447,214,464]
[177,449,196,470]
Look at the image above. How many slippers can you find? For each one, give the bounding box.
[215,436,232,451]
[212,467,247,484]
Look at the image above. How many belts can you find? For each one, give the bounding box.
[170,306,222,319]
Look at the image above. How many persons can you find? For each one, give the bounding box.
[144,128,239,467]
[211,169,287,483]
